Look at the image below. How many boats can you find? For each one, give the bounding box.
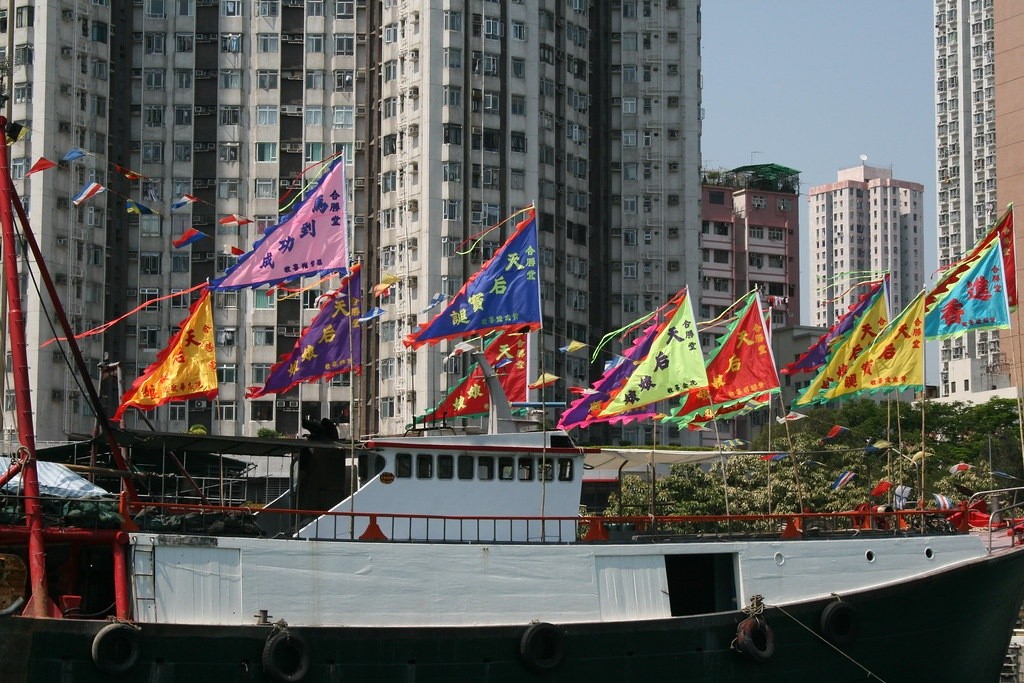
[0,115,1024,683]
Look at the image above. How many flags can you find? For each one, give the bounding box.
[223,243,244,257]
[724,439,746,447]
[357,307,386,321]
[375,274,398,298]
[419,292,449,314]
[71,181,106,205]
[26,157,58,178]
[570,386,585,394]
[528,373,558,390]
[219,214,253,228]
[173,228,207,249]
[759,411,1017,509]
[125,199,160,215]
[126,171,151,181]
[62,146,90,163]
[559,341,586,354]
[496,357,513,369]
[734,400,763,422]
[6,124,31,146]
[173,191,198,209]
